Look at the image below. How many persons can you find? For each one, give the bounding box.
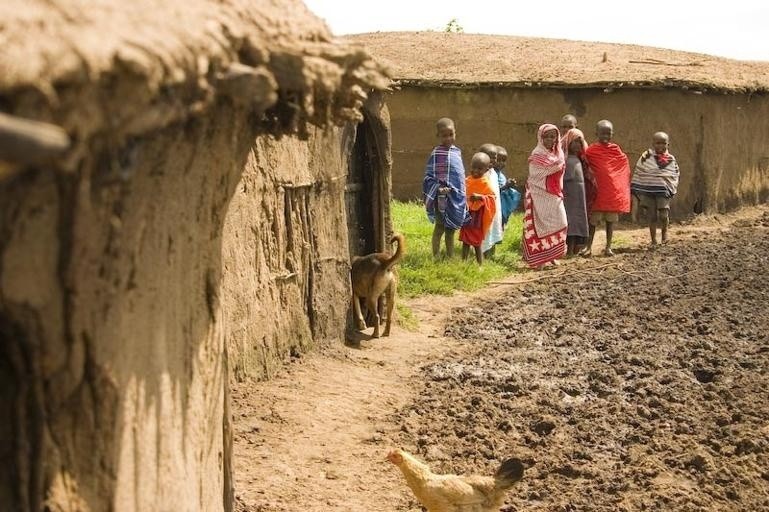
[422,114,679,267]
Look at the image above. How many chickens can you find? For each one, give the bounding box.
[385,447,523,512]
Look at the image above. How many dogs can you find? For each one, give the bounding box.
[350,233,405,339]
